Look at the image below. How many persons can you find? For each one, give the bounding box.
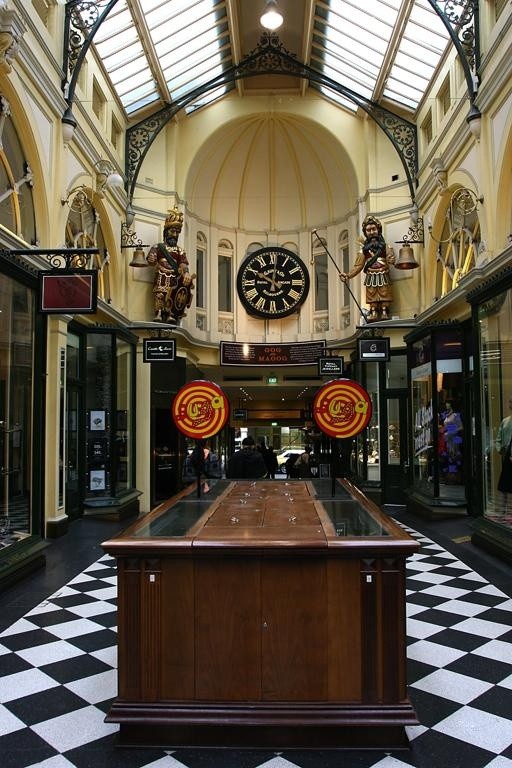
[204,480,218,496]
[495,397,511,516]
[182,439,222,484]
[338,224,396,320]
[147,225,187,323]
[443,400,463,472]
[224,437,313,479]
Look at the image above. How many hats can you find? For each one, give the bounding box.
[242,436,255,445]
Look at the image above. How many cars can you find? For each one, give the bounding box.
[274,446,313,473]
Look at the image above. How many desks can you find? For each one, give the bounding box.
[98,476,424,754]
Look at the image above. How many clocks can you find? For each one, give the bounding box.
[236,246,310,320]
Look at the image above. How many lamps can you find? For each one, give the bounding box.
[92,159,124,187]
[259,0,285,31]
